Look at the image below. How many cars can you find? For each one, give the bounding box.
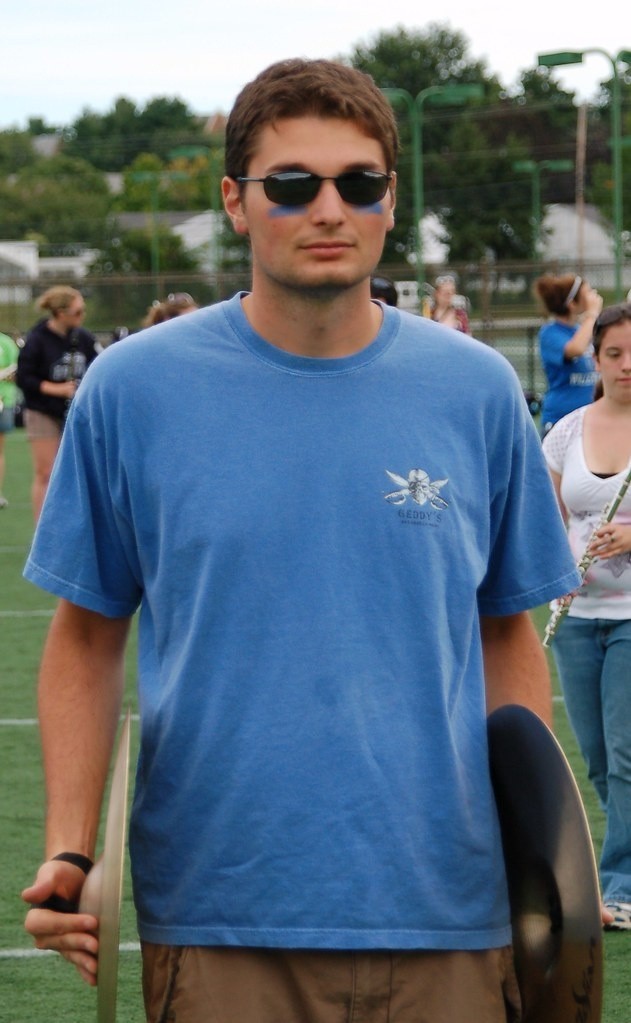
[395,279,470,316]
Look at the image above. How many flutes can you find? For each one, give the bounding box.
[542,468,631,648]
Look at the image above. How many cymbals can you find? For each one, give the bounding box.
[75,706,132,1023]
[485,702,607,1023]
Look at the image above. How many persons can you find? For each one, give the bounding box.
[540,303,631,930]
[537,274,604,441]
[0,333,25,508]
[430,279,470,335]
[146,293,198,326]
[370,277,398,306]
[16,286,100,523]
[23,59,615,1023]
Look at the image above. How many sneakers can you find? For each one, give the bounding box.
[0,497,9,508]
[602,901,631,930]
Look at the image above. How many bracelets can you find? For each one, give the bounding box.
[51,853,93,875]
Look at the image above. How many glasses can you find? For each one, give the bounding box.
[67,307,87,318]
[235,171,393,205]
